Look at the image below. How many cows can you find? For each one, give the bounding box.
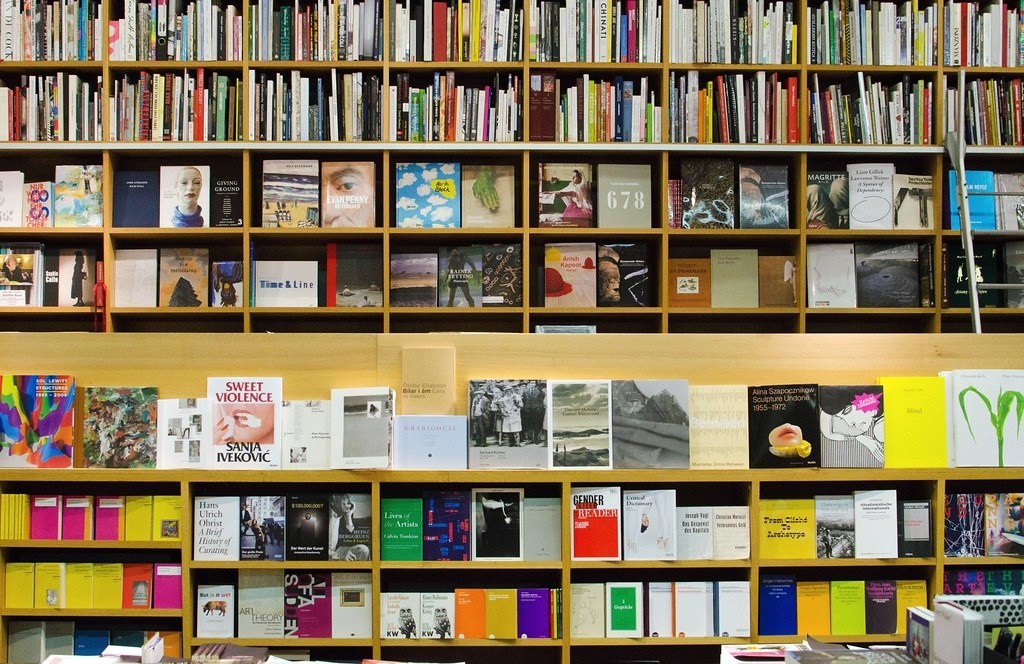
[202,600,228,616]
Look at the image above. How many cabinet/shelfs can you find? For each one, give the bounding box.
[0,0,1024,664]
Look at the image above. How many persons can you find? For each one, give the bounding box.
[4,256,24,282]
[246,519,267,559]
[321,161,376,228]
[543,170,594,219]
[240,504,252,536]
[169,167,207,227]
[360,294,375,307]
[442,247,481,307]
[343,285,353,296]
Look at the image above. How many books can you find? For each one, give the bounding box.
[0,0,243,143]
[390,156,1024,317]
[249,157,385,307]
[0,161,244,308]
[529,0,801,144]
[806,0,1024,147]
[247,0,525,142]
[0,367,1024,664]
